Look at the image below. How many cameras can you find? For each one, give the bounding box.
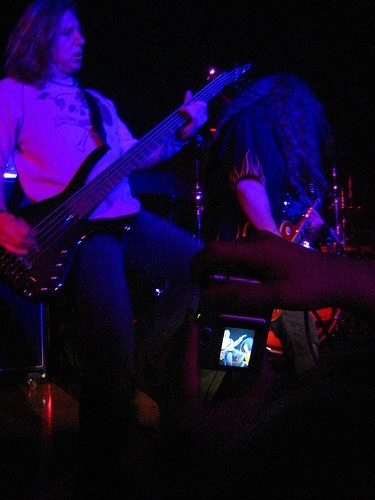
[195,276,268,373]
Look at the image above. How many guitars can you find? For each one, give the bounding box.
[242,199,341,355]
[0,62,256,305]
